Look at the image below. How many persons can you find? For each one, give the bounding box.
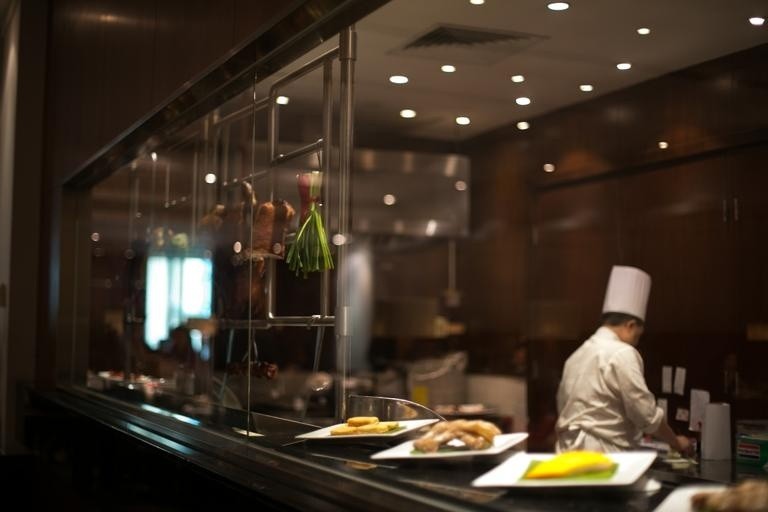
[555,310,696,461]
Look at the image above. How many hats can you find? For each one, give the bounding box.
[601,265,652,323]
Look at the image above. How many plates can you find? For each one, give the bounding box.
[369,430,530,465]
[650,485,743,512]
[470,449,659,492]
[293,417,440,442]
